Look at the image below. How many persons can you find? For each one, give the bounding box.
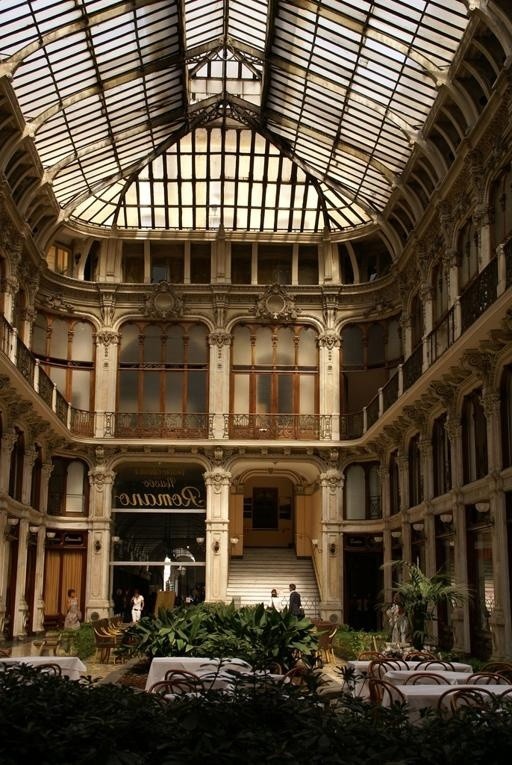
[192,583,201,603]
[267,589,282,611]
[131,590,145,624]
[288,583,301,615]
[64,589,81,631]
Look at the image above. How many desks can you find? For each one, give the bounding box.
[1,657,87,681]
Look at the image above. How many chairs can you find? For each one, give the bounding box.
[147,656,306,694]
[348,652,512,724]
[93,616,132,662]
[312,617,338,663]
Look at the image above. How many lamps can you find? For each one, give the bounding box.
[412,524,424,532]
[112,537,124,545]
[8,518,19,526]
[197,538,205,548]
[375,537,383,542]
[392,532,402,538]
[230,538,238,544]
[312,539,320,545]
[30,526,40,534]
[47,532,57,539]
[440,514,452,522]
